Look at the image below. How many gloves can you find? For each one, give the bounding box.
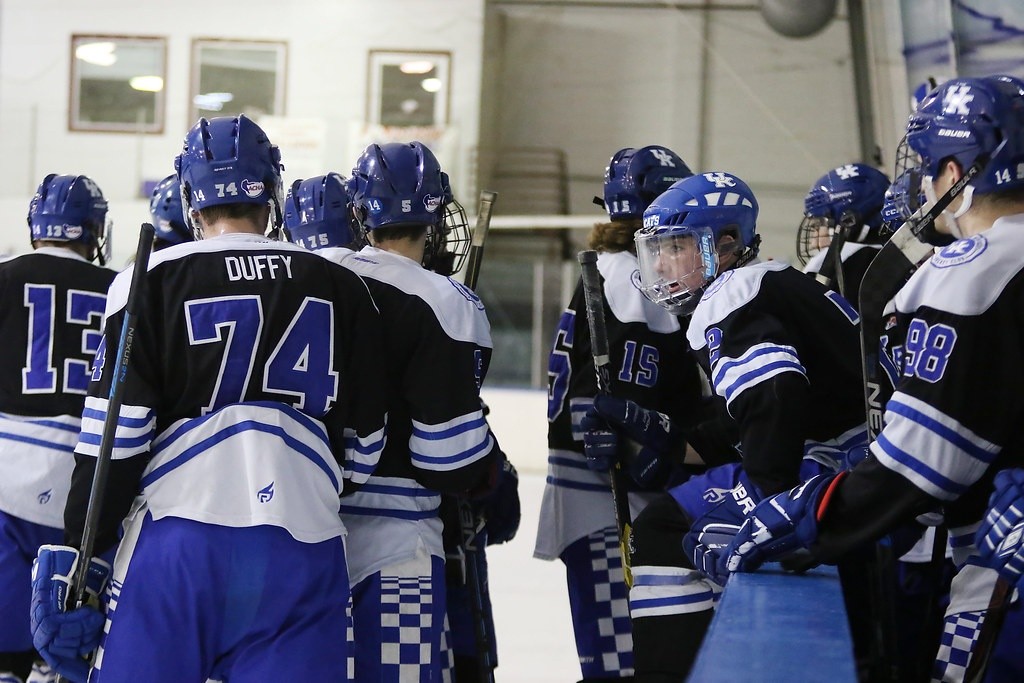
[477,451,520,546]
[29,545,111,683]
[796,443,868,486]
[720,473,847,584]
[683,469,766,582]
[581,395,684,500]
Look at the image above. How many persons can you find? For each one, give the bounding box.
[0,115,523,681]
[533,77,1024,683]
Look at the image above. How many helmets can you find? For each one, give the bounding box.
[631,171,760,314]
[26,174,113,265]
[285,172,365,252]
[880,167,925,234]
[423,172,471,277]
[174,113,281,230]
[345,141,442,249]
[593,146,693,222]
[150,173,194,244]
[796,163,891,269]
[894,74,1023,243]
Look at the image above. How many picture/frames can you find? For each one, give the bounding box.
[68,33,167,134]
[188,38,286,152]
[366,49,452,150]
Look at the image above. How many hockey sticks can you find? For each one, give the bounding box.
[577,244,636,588]
[70,218,156,610]
[814,223,851,286]
[463,187,499,291]
[855,200,935,444]
